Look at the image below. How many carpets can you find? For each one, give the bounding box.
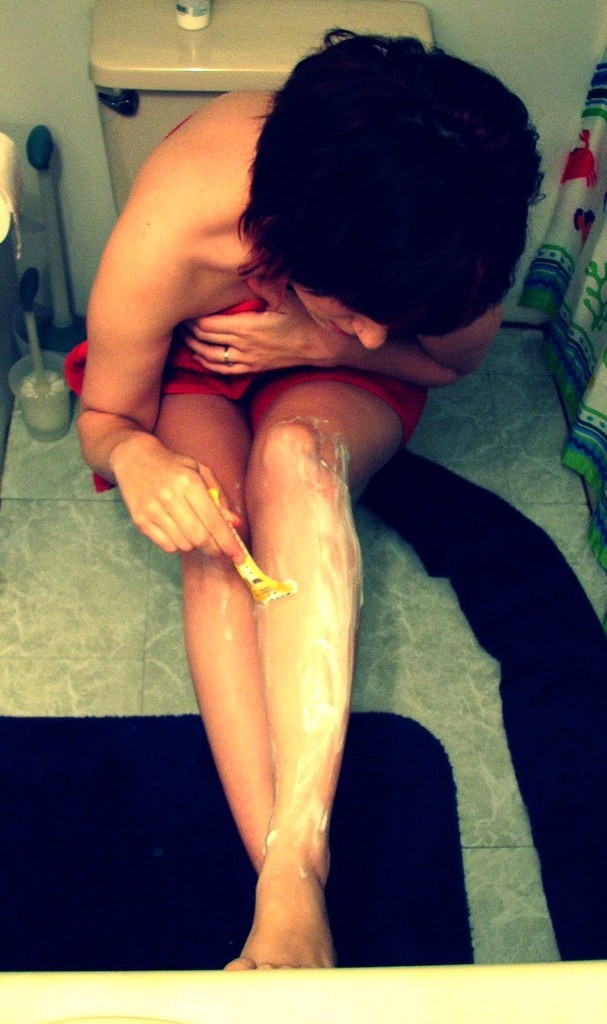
[0,711,478,971]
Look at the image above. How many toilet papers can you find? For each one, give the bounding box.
[0,131,26,263]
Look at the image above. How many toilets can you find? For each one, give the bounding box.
[87,1,435,218]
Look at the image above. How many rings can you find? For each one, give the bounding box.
[224,346,229,364]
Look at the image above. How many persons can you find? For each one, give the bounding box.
[67,26,546,972]
